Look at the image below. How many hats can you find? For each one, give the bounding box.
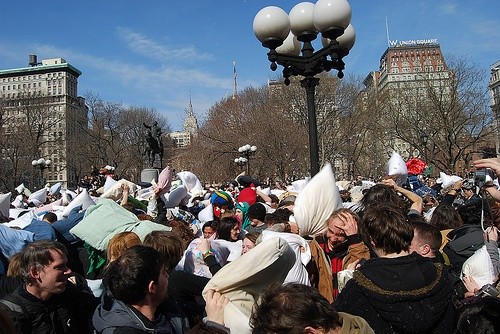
[246,203,266,222]
[281,196,295,204]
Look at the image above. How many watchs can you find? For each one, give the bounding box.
[285,223,291,231]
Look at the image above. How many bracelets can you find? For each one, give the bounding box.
[453,188,460,192]
[202,250,213,259]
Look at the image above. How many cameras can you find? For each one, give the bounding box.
[474,168,494,189]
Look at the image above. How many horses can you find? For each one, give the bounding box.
[142,122,164,170]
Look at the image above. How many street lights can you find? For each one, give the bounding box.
[238,144,258,176]
[234,157,248,173]
[253,0,356,179]
[31,158,52,189]
[104,164,115,179]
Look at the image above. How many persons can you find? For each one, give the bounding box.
[143,121,161,151]
[0,158,500,334]
